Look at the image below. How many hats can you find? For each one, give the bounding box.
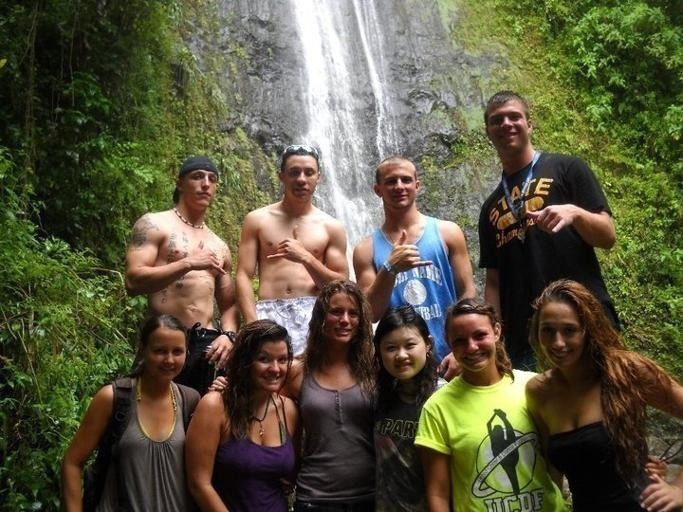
[173,155,220,204]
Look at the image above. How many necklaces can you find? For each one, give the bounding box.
[385,216,421,243]
[252,392,272,437]
[136,377,178,442]
[173,207,206,230]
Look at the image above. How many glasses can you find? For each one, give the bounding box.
[283,144,319,156]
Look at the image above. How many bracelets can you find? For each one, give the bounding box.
[383,260,399,277]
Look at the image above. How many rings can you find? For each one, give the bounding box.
[419,260,426,266]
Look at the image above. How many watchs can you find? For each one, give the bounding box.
[224,330,236,343]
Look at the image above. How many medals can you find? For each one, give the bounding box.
[517,227,526,240]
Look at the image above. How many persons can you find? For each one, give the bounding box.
[61,314,200,512]
[208,279,376,512]
[235,143,350,358]
[352,156,477,383]
[366,301,450,512]
[525,279,683,512]
[185,318,299,512]
[478,89,621,372]
[412,293,667,512]
[125,154,241,398]
